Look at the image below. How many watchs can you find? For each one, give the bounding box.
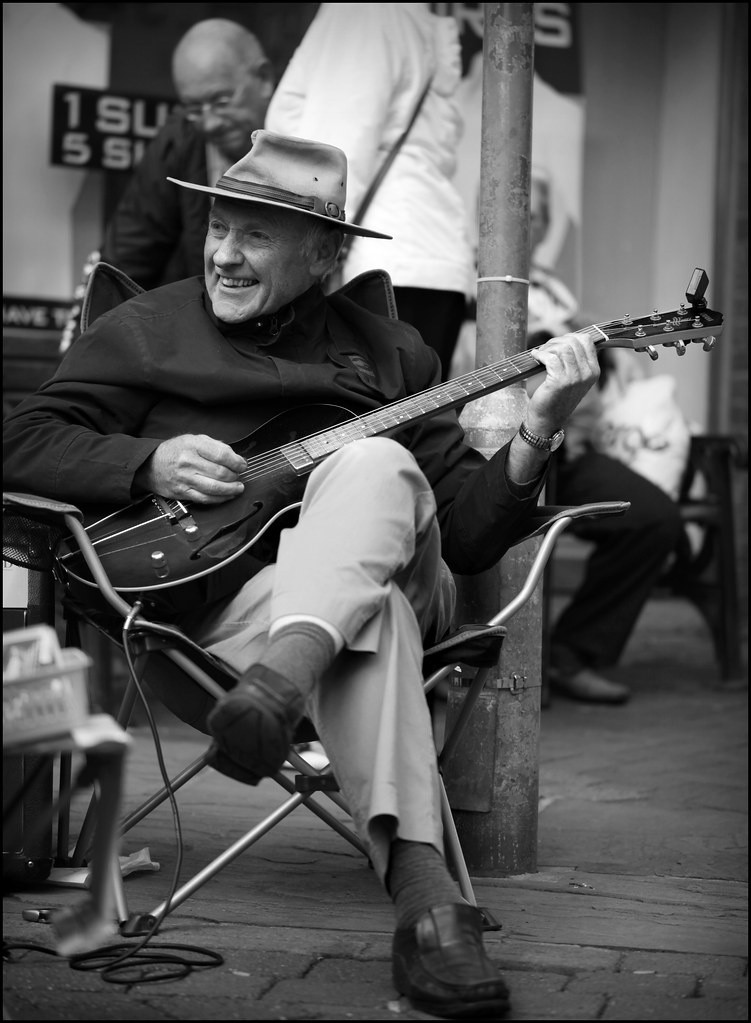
[518,419,566,452]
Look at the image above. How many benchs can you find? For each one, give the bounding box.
[568,433,748,690]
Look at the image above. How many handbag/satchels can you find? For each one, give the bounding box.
[593,374,688,500]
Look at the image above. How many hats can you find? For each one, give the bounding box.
[165,131,392,240]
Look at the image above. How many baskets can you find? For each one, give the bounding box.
[2,622,92,747]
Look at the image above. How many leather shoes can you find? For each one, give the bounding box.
[392,902,511,1021]
[203,664,303,784]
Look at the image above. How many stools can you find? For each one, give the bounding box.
[3,734,128,955]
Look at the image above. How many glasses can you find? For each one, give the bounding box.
[176,65,256,123]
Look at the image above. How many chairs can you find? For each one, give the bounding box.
[3,262,630,938]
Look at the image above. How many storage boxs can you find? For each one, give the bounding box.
[3,646,94,747]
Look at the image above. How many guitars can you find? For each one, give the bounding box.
[42,263,729,657]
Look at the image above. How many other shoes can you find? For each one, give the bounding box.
[547,666,629,704]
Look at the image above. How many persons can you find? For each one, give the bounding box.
[87,2,685,709]
[1,128,603,1021]
